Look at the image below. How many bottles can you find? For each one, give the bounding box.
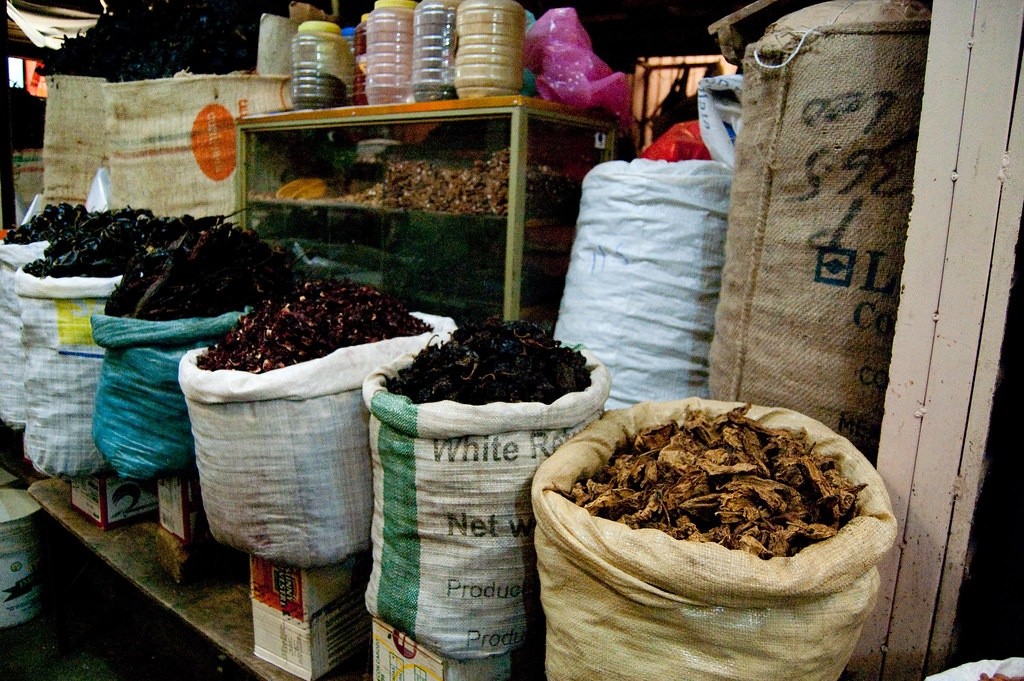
[342,0,524,105]
[289,20,351,109]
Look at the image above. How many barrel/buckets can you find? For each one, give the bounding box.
[0,486,45,627]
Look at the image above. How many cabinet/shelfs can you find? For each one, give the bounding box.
[237,96,613,381]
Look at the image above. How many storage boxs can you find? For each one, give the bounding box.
[71,439,534,681]
